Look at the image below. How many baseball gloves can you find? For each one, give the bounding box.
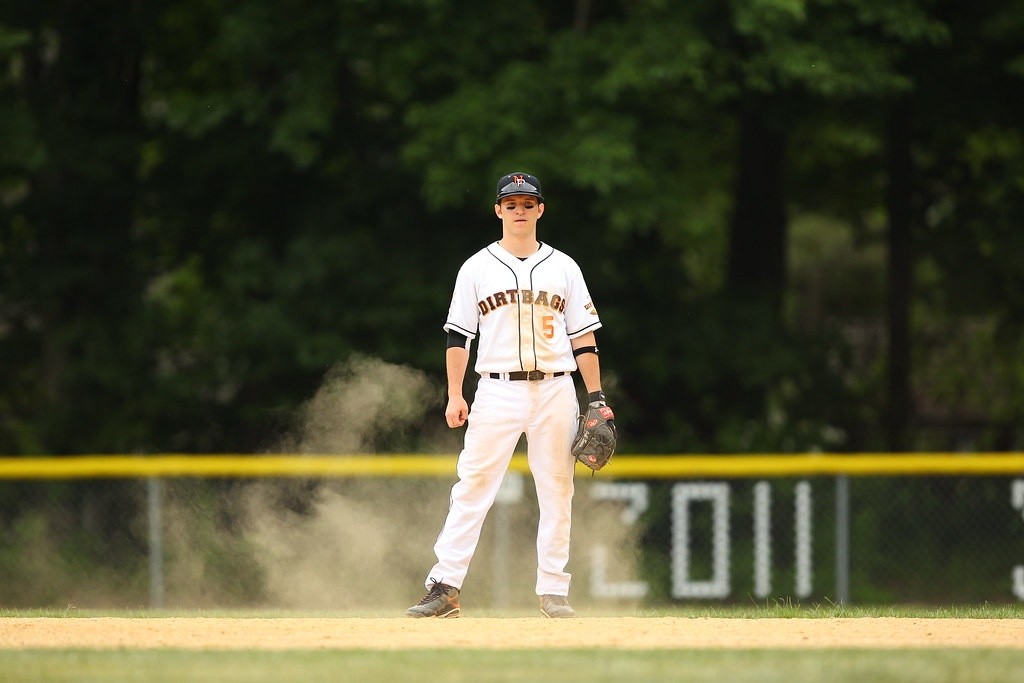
[571,402,618,479]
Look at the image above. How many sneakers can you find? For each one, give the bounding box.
[406,577,460,619]
[539,594,576,618]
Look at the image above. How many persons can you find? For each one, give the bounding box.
[405,172,617,619]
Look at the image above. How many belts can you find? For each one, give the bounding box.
[490,370,564,381]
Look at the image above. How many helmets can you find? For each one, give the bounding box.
[497,172,544,205]
